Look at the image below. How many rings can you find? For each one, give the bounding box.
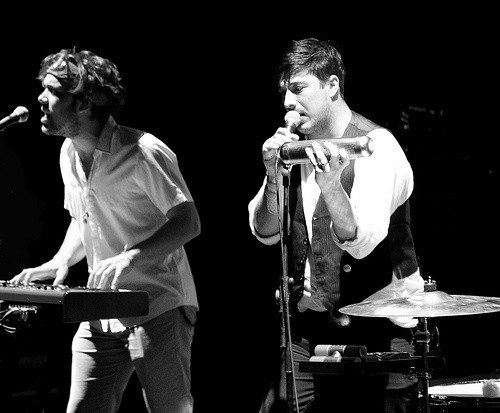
[319,161,328,166]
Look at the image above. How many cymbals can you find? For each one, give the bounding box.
[338,287,500,320]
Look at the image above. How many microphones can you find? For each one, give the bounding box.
[276,111,301,163]
[0,106,29,131]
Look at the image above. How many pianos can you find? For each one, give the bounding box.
[1,279,151,335]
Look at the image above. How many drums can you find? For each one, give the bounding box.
[416,377,500,413]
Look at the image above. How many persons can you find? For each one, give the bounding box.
[8,46,201,413]
[248,37,425,413]
